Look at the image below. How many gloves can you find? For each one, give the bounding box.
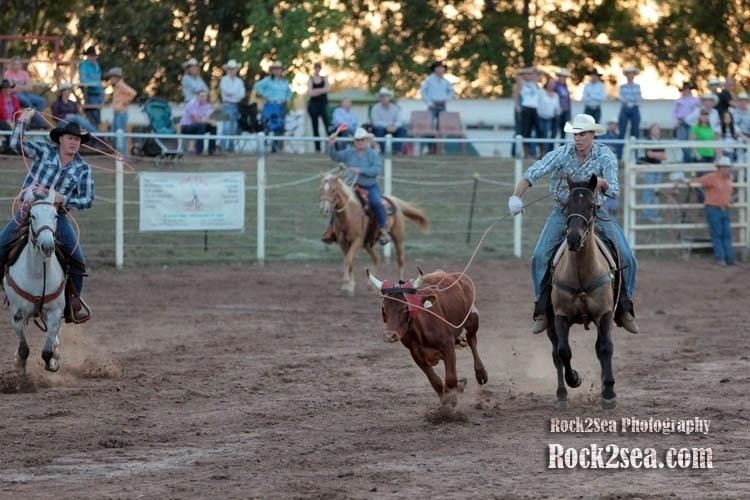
[509,196,525,216]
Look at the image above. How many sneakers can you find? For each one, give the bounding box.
[65,311,87,322]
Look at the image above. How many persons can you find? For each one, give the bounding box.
[0,107,94,327]
[690,155,742,267]
[321,122,393,246]
[506,113,641,334]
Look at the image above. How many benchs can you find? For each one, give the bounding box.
[2,33,118,152]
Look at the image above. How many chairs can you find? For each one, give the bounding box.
[201,111,470,154]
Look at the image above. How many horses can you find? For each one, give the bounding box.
[1,184,68,378]
[545,172,616,410]
[318,169,429,296]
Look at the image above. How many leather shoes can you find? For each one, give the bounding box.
[532,315,546,334]
[621,312,639,333]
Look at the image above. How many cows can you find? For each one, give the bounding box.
[365,265,489,422]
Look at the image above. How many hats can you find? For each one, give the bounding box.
[624,66,640,75]
[679,81,719,105]
[431,62,447,71]
[55,47,122,95]
[715,157,732,167]
[350,128,374,141]
[50,123,88,143]
[375,87,394,99]
[586,69,602,76]
[563,114,607,136]
[0,80,15,89]
[182,59,283,68]
